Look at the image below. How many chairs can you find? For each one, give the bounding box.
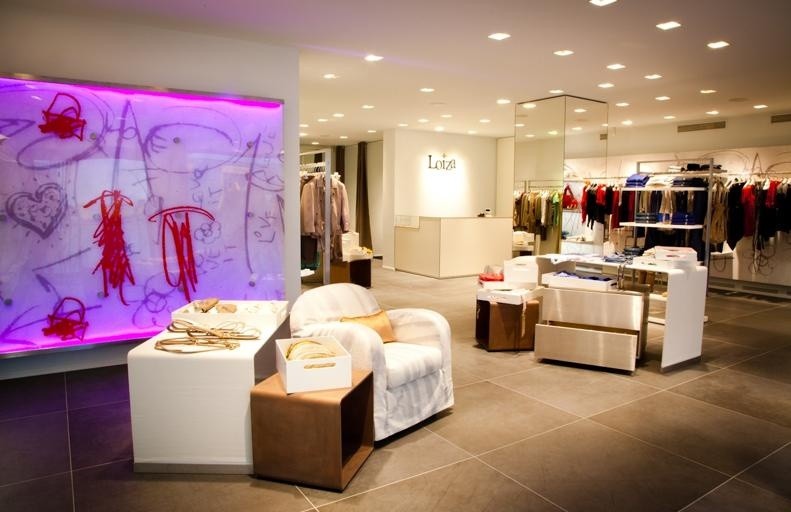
[289,281,454,441]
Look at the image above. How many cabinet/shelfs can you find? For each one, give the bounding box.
[617,158,728,324]
[534,283,650,371]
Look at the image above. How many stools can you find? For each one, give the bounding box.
[476,300,539,352]
[251,366,374,491]
[330,260,371,288]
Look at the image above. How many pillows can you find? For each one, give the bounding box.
[341,308,396,343]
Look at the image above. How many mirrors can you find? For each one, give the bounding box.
[511,95,565,258]
[558,96,609,255]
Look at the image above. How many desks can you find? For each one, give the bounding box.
[127,312,291,475]
[533,255,707,372]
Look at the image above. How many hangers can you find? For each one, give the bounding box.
[728,172,791,184]
[154,320,260,350]
